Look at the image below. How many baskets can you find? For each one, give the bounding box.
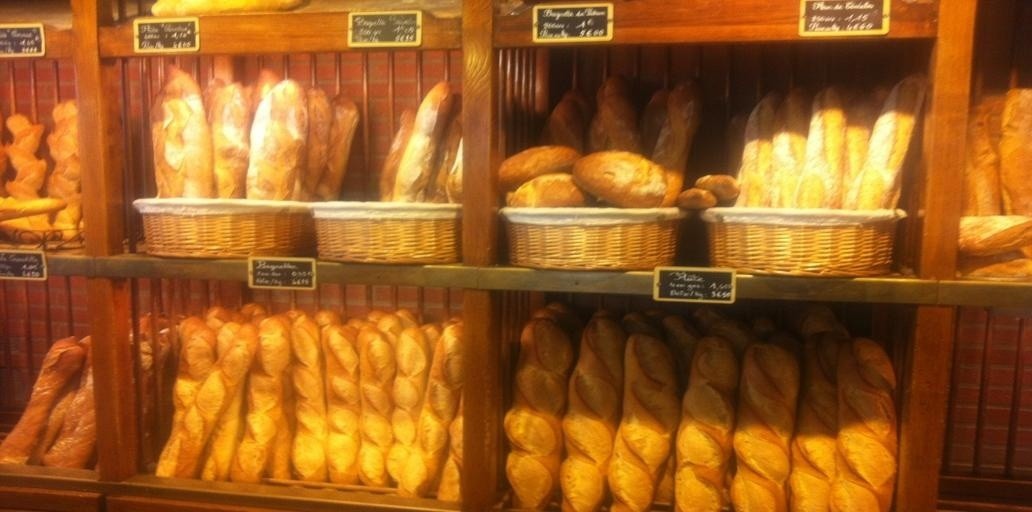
[129,195,315,264]
[306,197,465,269]
[496,204,694,275]
[700,206,910,284]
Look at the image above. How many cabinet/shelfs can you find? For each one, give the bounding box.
[1,1,1032,511]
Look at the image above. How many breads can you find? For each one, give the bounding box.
[0,311,171,467]
[956,85,1032,279]
[0,97,86,243]
[497,63,701,213]
[149,67,359,202]
[726,62,927,211]
[504,302,899,512]
[154,303,463,503]
[695,174,738,202]
[380,82,462,203]
[677,188,718,210]
